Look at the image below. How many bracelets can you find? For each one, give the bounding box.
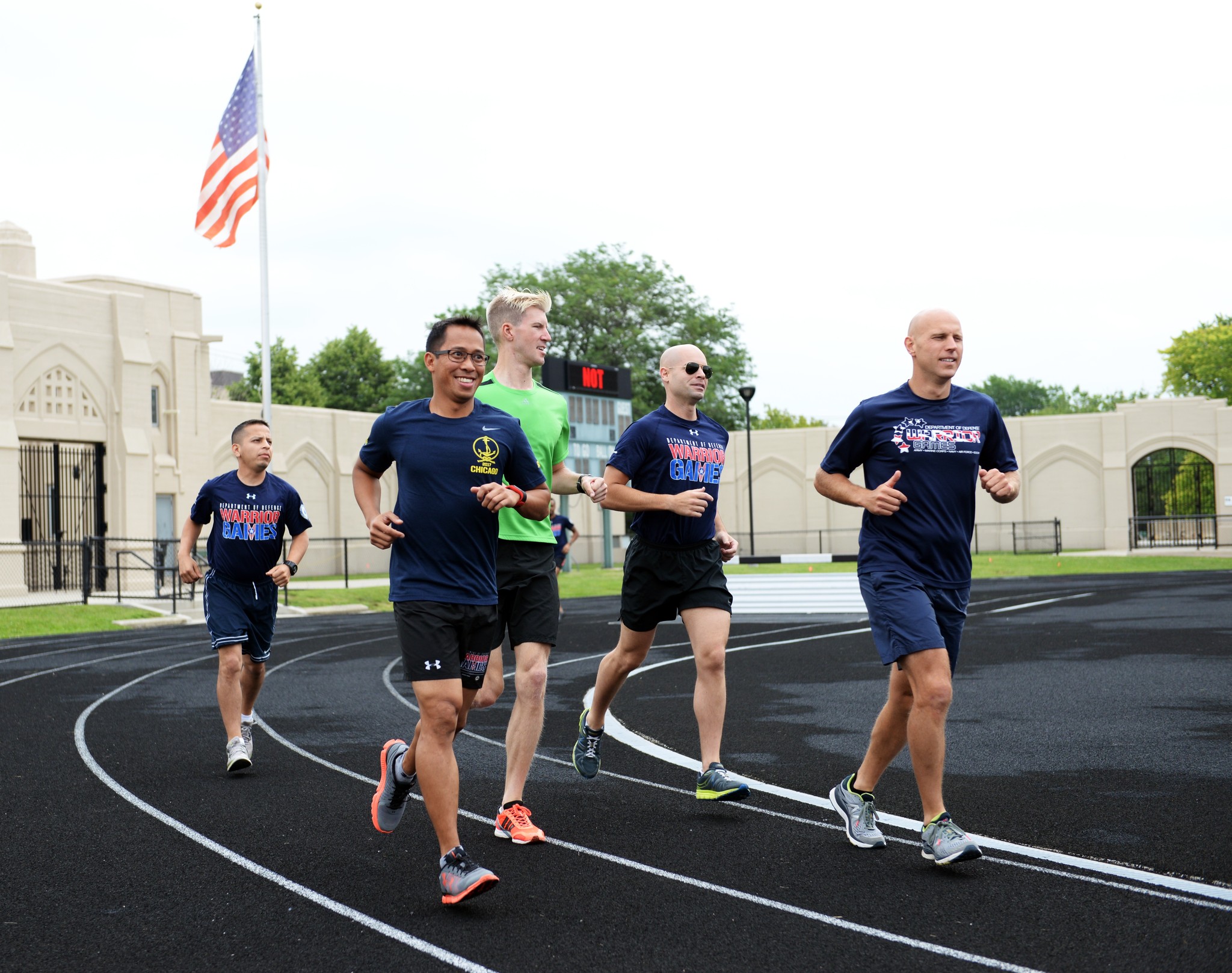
[576,472,586,493]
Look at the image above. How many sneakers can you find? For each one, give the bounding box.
[921,811,983,865]
[241,717,257,760]
[573,707,605,779]
[495,800,546,845]
[696,762,750,801]
[439,844,500,904]
[226,736,252,772]
[829,773,887,849]
[371,739,417,833]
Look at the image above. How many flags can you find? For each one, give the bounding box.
[197,52,267,251]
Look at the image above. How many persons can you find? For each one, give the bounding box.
[177,419,312,772]
[472,283,608,845]
[548,496,579,618]
[813,309,1021,866]
[352,315,551,904]
[572,342,751,801]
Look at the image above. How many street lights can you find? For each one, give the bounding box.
[737,386,756,555]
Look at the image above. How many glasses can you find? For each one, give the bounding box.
[666,362,713,379]
[430,349,490,366]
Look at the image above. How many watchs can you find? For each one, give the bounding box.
[503,482,528,507]
[284,559,299,576]
